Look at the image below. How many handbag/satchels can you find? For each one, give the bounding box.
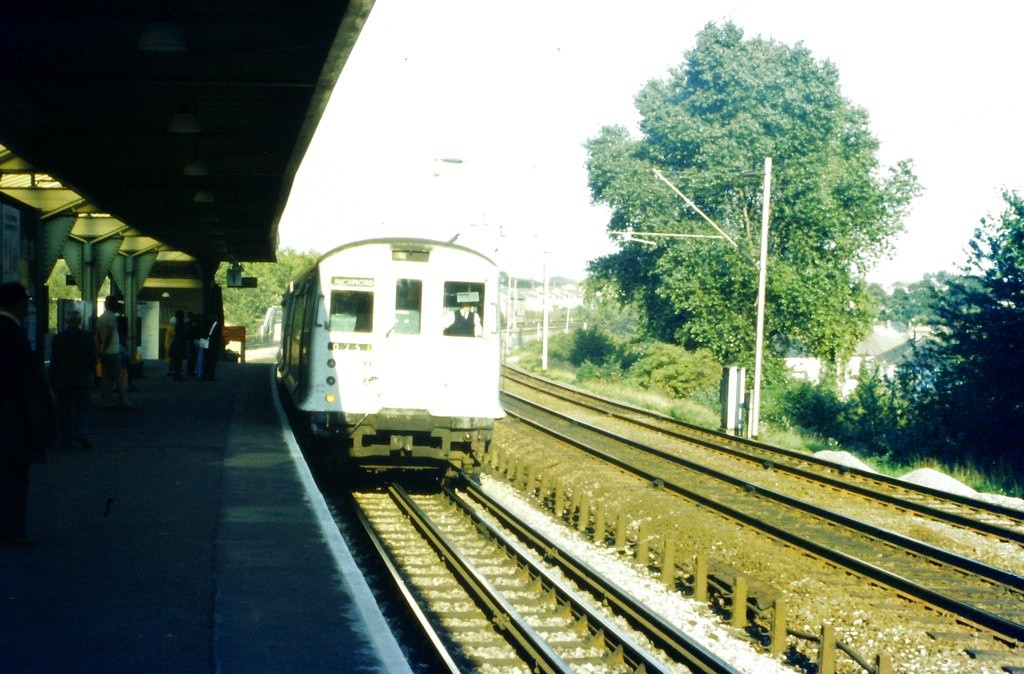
[199,338,210,349]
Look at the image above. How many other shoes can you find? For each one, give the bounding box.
[76,432,92,449]
[0,532,38,548]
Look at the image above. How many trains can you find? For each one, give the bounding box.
[275,235,508,487]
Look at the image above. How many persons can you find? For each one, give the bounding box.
[165,308,223,383]
[443,300,484,337]
[0,281,39,548]
[49,310,97,451]
[94,295,141,411]
